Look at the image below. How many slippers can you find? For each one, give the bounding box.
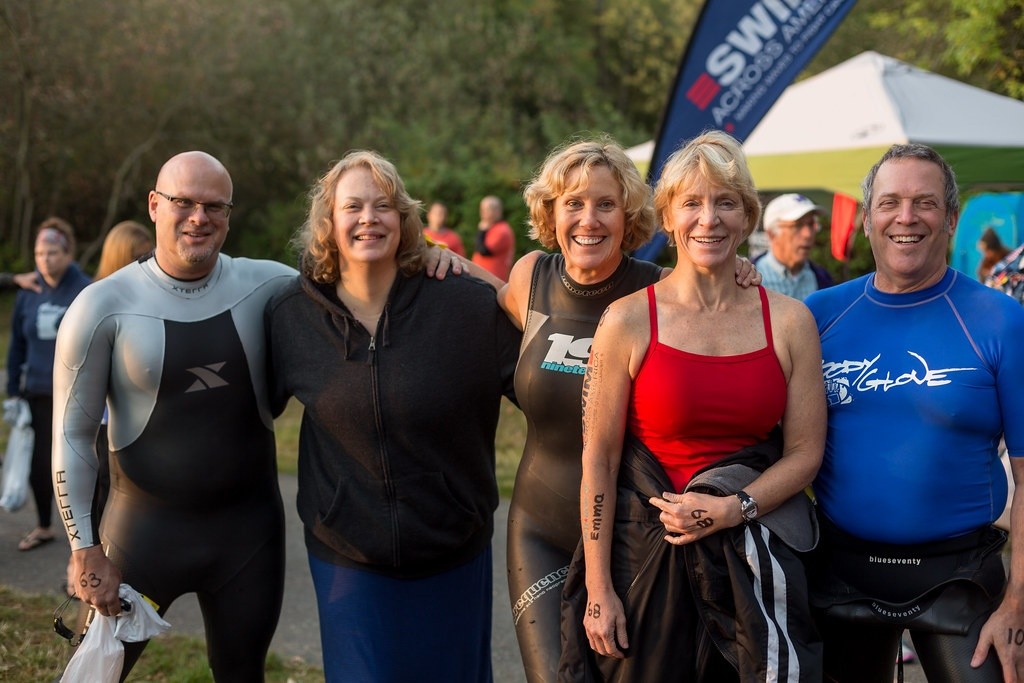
[17,534,55,552]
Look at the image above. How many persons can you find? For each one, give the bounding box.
[447,129,763,683]
[470,195,518,284]
[265,150,525,683]
[748,191,836,302]
[64,220,155,600]
[0,271,43,296]
[562,130,827,683]
[975,227,1014,284]
[5,217,91,551]
[51,151,472,683]
[801,142,1024,683]
[420,198,467,258]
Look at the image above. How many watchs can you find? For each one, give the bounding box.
[736,490,759,525]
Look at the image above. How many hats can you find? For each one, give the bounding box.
[763,193,827,230]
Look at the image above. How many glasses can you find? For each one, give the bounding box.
[157,192,233,217]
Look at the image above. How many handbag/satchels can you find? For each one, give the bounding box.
[1,400,34,514]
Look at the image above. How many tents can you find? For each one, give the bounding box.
[619,49,1023,292]
